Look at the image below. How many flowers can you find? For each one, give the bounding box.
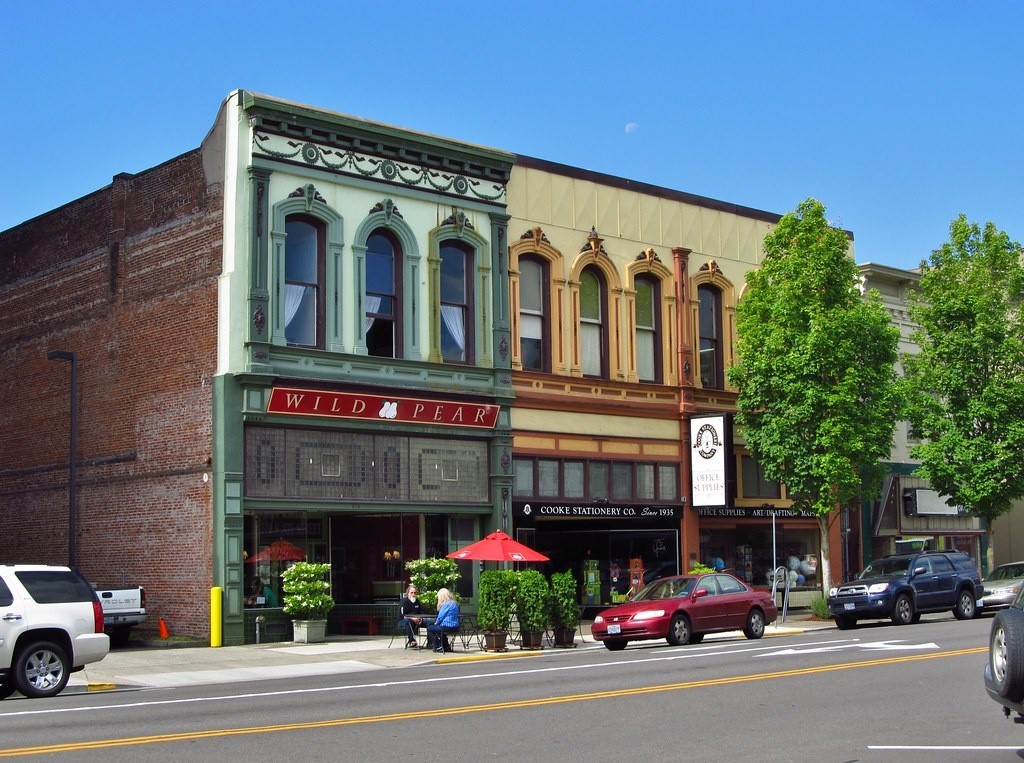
[280,562,335,619]
[404,558,462,615]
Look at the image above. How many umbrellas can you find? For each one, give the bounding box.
[245,538,309,566]
[446,529,550,571]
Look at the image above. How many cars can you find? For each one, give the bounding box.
[976,561,1024,619]
[590,572,777,651]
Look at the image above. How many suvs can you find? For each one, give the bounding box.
[826,549,984,630]
[984,578,1024,725]
[0,562,110,700]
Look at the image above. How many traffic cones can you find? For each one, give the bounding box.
[159,616,168,640]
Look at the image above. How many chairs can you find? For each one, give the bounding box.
[388,608,467,654]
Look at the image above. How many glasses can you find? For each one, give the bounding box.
[408,592,417,594]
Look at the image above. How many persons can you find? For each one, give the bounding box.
[397,585,435,648]
[427,587,459,652]
[244,577,278,609]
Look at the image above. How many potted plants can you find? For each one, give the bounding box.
[477,568,580,652]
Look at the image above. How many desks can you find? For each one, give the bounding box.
[406,611,515,652]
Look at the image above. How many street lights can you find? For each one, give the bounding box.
[761,502,778,608]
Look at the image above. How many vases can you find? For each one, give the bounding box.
[418,628,428,647]
[291,619,327,643]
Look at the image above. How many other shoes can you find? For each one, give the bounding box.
[426,643,435,648]
[433,646,443,653]
[444,645,450,652]
[409,640,417,648]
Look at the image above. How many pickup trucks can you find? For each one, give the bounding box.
[94,585,147,649]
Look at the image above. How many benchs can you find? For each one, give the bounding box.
[339,616,383,635]
